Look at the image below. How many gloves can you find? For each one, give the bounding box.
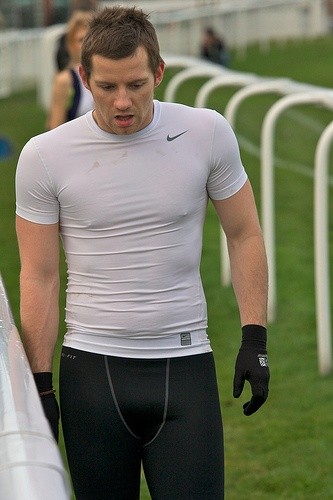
[33,371,60,445]
[232,324,271,416]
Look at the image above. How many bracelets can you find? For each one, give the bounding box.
[37,390,57,395]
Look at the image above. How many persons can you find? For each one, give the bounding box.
[49,9,96,130]
[199,24,231,67]
[15,4,270,500]
